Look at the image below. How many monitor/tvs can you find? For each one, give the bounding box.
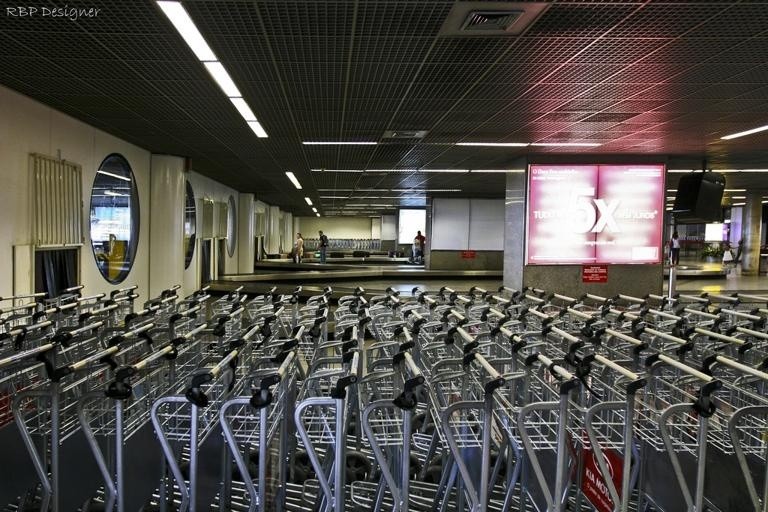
[673,171,726,225]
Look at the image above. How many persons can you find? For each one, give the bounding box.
[670,230,682,266]
[413,231,425,250]
[315,230,328,264]
[292,233,304,264]
[663,240,670,254]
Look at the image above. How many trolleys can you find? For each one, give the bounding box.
[0,285,768,512]
